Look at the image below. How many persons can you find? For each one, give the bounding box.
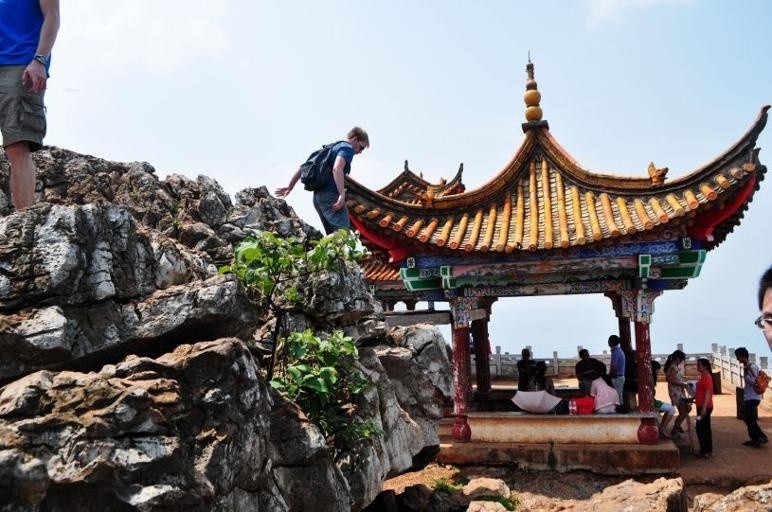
[650,360,675,438]
[694,357,715,459]
[625,350,639,409]
[662,349,693,434]
[755,264,772,353]
[516,348,538,391]
[607,334,630,413]
[1,1,62,209]
[527,361,556,416]
[567,380,595,414]
[734,347,769,447]
[274,126,370,236]
[588,369,622,414]
[576,348,607,382]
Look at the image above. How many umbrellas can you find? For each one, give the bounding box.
[512,380,564,414]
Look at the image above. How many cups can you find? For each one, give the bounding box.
[568,400,577,416]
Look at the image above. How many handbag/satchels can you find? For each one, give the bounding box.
[754,370,768,394]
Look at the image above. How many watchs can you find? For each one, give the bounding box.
[33,53,49,67]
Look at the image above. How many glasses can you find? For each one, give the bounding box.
[359,141,365,151]
[754,315,772,328]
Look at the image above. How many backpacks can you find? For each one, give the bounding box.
[300,141,350,191]
